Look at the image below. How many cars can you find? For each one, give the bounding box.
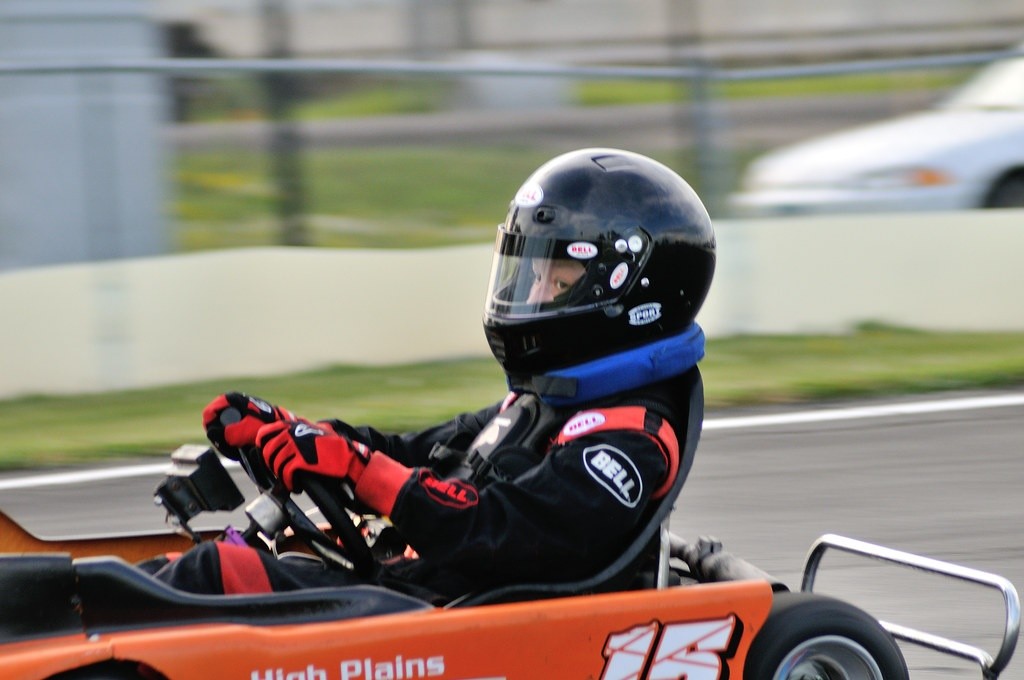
[730,53,1024,212]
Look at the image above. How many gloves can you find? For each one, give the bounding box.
[256,414,364,493]
[202,394,321,458]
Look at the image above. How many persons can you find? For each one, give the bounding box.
[132,143,718,599]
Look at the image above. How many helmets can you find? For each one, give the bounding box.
[486,149,721,371]
